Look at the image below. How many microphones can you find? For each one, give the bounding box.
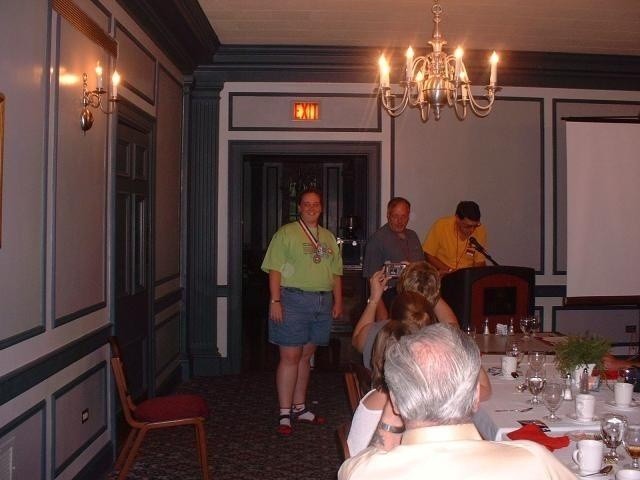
[469,237,490,256]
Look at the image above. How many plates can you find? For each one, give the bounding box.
[571,465,614,480]
[566,413,603,426]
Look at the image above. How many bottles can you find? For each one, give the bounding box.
[581,368,590,393]
[563,374,573,400]
[483,319,490,335]
[508,320,514,335]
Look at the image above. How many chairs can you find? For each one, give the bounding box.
[339,362,374,417]
[331,422,367,461]
[109,339,211,480]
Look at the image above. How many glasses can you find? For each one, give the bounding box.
[459,220,483,230]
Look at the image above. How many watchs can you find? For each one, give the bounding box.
[270,299,281,302]
[368,299,379,305]
[376,422,406,433]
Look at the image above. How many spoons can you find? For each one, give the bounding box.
[582,465,613,478]
[496,407,533,412]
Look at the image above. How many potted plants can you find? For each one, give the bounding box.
[557,332,611,392]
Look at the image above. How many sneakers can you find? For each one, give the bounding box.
[275,415,292,439]
[294,410,328,426]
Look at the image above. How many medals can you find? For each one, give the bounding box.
[297,218,323,264]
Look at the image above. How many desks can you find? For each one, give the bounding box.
[441,266,535,332]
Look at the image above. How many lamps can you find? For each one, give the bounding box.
[375,6,504,123]
[78,63,124,136]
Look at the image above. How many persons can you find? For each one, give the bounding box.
[363,197,425,322]
[261,189,343,436]
[347,321,423,455]
[351,260,461,372]
[391,292,493,403]
[336,323,577,479]
[423,201,489,274]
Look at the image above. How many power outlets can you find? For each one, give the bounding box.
[625,325,636,332]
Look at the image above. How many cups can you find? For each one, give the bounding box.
[527,351,546,377]
[520,314,533,338]
[463,321,476,339]
[573,440,603,476]
[575,394,596,422]
[600,414,628,462]
[526,366,546,408]
[542,384,564,422]
[506,339,524,372]
[530,316,538,336]
[622,424,640,469]
[613,383,633,407]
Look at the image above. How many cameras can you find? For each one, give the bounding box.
[383,262,406,278]
